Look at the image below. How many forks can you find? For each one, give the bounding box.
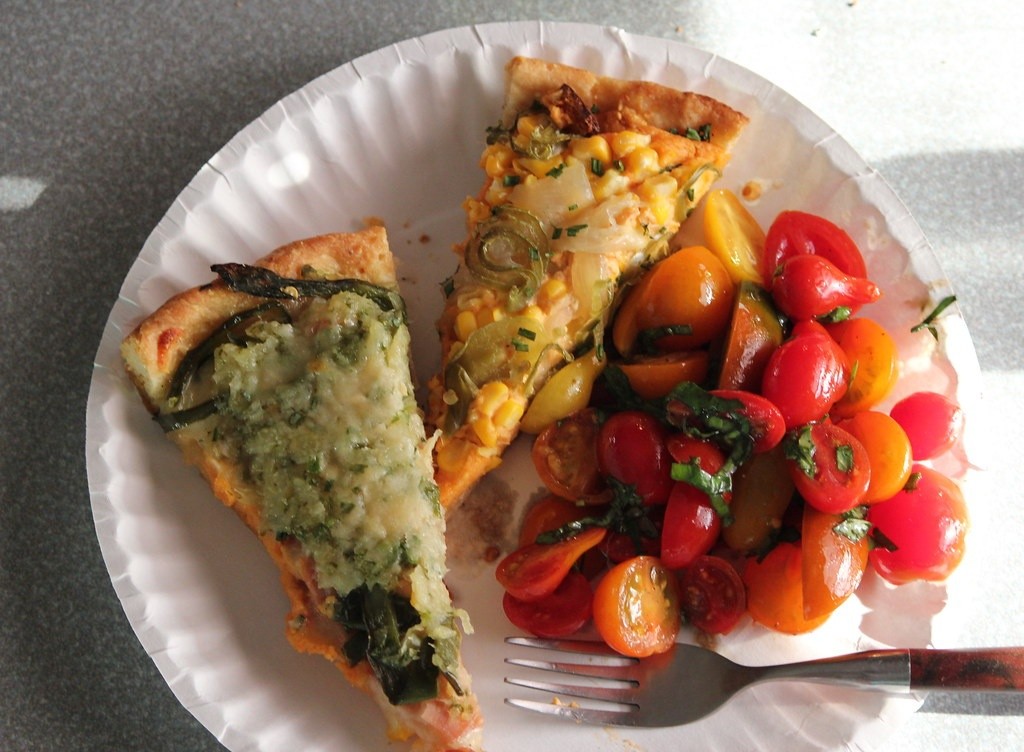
[504,634,1024,731]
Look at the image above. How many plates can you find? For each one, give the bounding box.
[85,22,989,752]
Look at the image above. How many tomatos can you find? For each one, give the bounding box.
[488,183,975,663]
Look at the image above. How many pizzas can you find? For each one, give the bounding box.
[118,57,758,752]
